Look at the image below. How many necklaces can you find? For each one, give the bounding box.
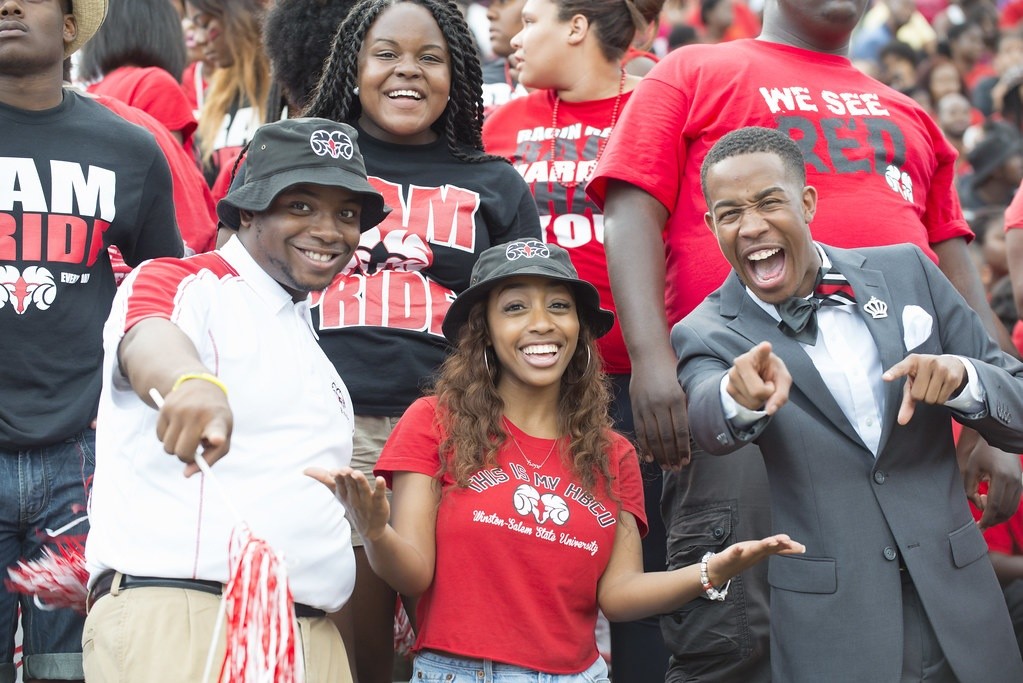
[502,416,560,469]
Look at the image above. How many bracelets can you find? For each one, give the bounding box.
[701,551,731,601]
[172,371,228,396]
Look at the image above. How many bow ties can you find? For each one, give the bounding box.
[770,264,878,348]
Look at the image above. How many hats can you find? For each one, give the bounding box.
[215,119,399,234]
[65,0,111,65]
[439,238,615,343]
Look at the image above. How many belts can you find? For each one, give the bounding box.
[87,569,329,619]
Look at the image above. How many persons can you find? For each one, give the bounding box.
[76,0,273,148]
[584,0,1023,683]
[0,0,184,683]
[463,0,762,683]
[850,0,1023,360]
[80,114,391,683]
[670,126,1023,683]
[264,0,544,683]
[305,237,806,683]
